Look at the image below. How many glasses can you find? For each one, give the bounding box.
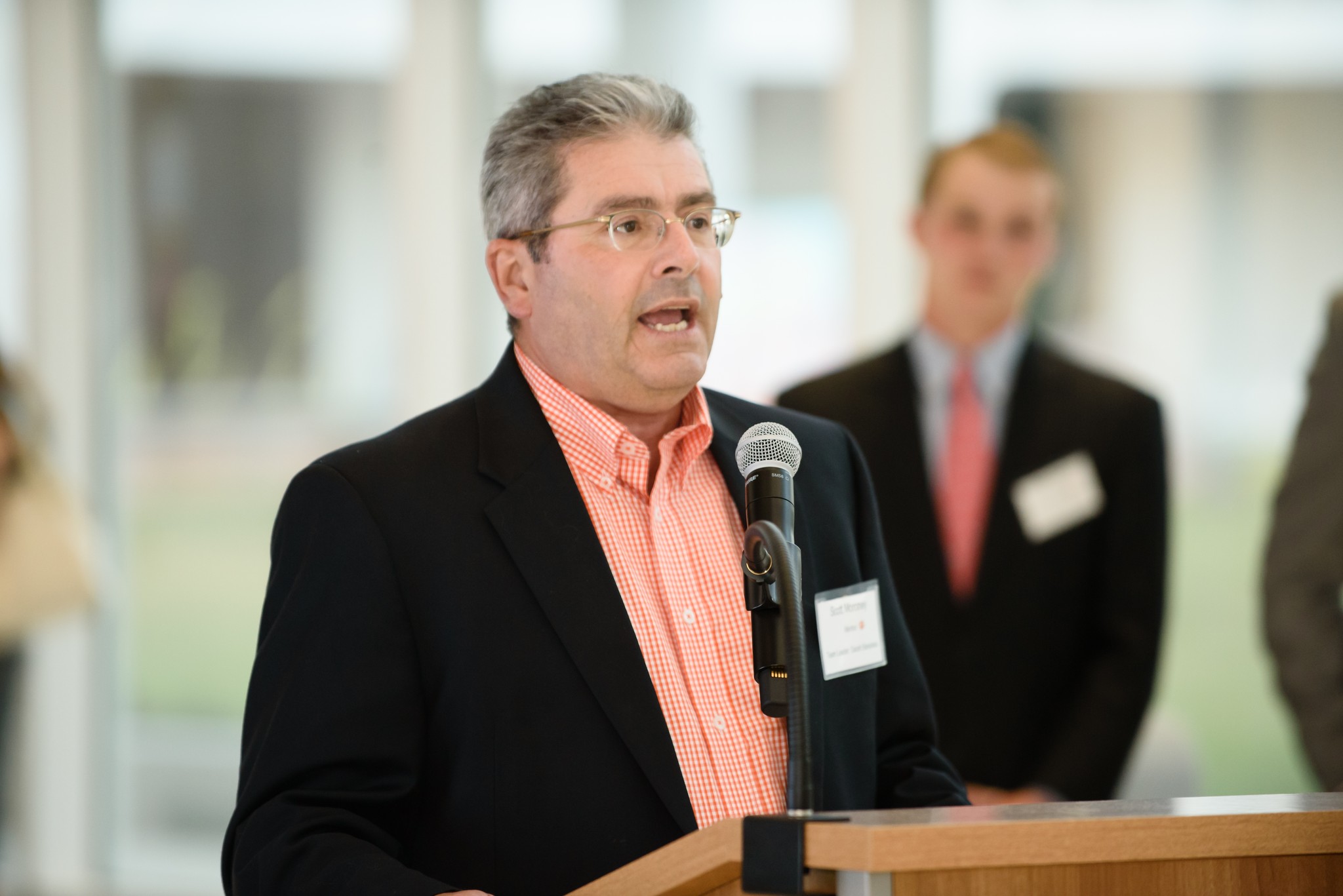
[503,206,741,259]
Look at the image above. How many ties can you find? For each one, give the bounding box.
[942,363,997,588]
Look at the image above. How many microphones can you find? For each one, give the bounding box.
[736,423,801,718]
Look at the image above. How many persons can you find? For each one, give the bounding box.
[777,125,1166,806]
[222,75,973,896]
[1262,292,1343,792]
